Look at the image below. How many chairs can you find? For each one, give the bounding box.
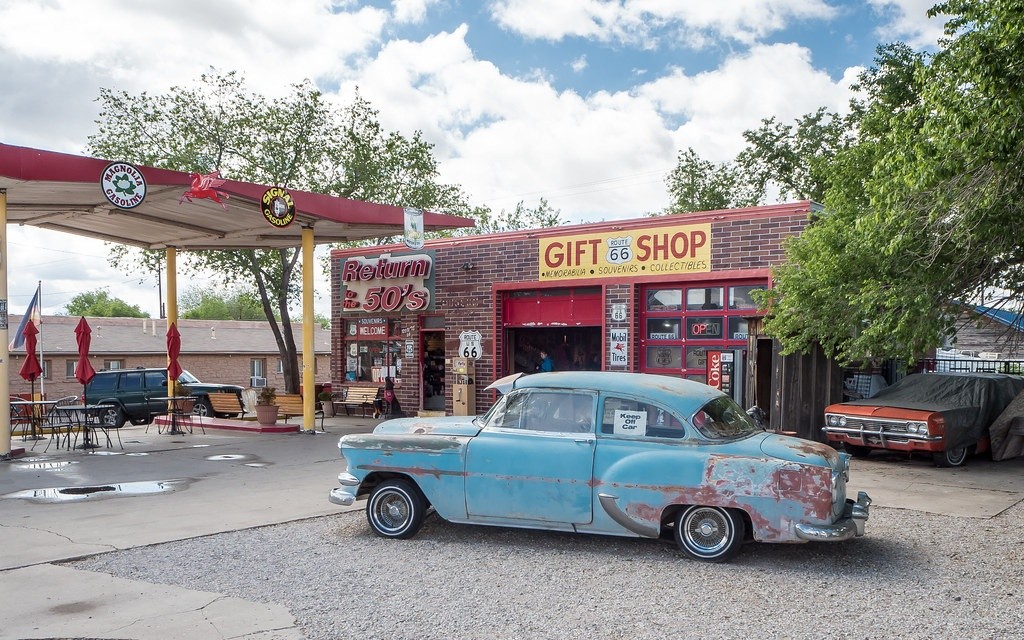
[145,396,206,434]
[10,396,123,453]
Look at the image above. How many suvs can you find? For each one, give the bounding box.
[81,365,247,429]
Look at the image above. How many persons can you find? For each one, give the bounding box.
[539,350,554,373]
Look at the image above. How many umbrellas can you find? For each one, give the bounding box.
[166,321,183,430]
[74,316,97,445]
[19,318,43,436]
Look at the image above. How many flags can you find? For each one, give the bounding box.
[8,286,43,351]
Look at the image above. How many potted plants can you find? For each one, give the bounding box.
[175,383,196,412]
[255,387,281,424]
[320,393,332,415]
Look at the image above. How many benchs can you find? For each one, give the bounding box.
[333,387,380,419]
[273,394,325,431]
[208,392,248,420]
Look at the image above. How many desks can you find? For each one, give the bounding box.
[56,405,115,449]
[151,397,198,435]
[10,400,58,440]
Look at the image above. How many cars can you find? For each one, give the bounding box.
[820,371,1024,468]
[328,370,874,564]
